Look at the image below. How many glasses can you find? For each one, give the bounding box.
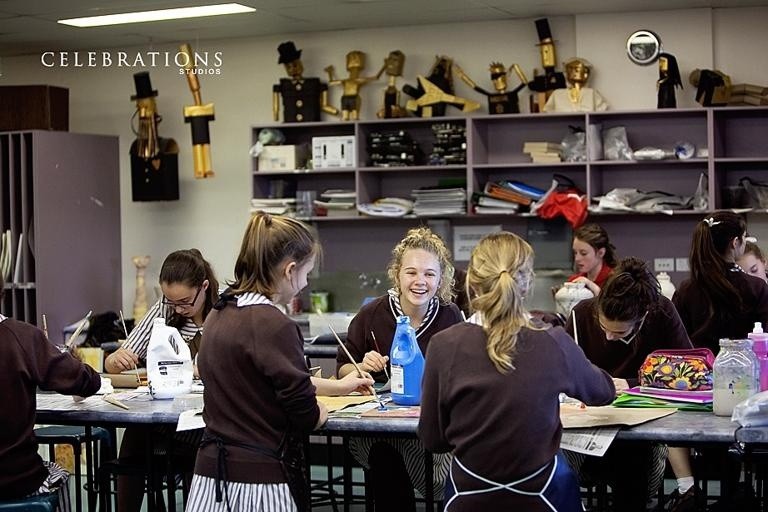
[161,288,201,307]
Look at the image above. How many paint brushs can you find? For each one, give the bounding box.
[371,331,390,380]
[328,323,385,408]
[119,310,142,386]
[61,310,92,353]
[571,310,578,346]
[42,314,48,340]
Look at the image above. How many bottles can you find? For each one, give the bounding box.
[653,272,677,302]
[554,282,594,321]
[712,322,768,416]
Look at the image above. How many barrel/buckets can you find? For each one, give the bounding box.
[145,317,194,402]
[388,316,425,407]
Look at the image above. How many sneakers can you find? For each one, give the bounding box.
[665,485,701,511]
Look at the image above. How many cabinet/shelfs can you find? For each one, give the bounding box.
[0,131,122,342]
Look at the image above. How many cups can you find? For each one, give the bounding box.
[296,190,315,217]
[309,290,329,312]
[286,294,303,315]
[79,348,107,373]
[171,394,203,415]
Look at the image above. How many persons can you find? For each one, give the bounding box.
[179,43,215,179]
[1,268,101,512]
[104,248,219,511]
[183,210,376,511]
[129,71,180,202]
[550,210,768,511]
[272,18,768,124]
[417,230,616,512]
[336,226,464,510]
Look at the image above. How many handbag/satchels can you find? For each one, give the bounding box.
[639,348,716,391]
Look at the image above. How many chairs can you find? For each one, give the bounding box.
[0,343,181,512]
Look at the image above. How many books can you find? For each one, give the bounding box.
[0,229,23,282]
[522,142,564,163]
[249,180,547,218]
